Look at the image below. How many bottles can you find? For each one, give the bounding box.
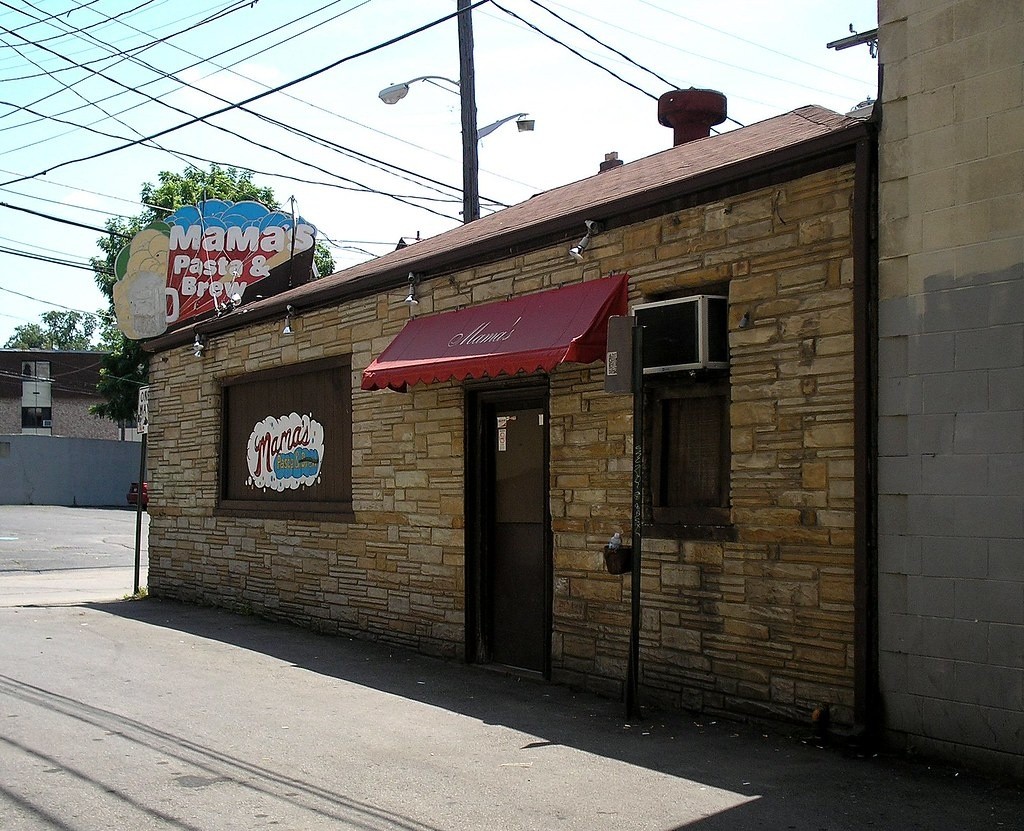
[609,533,621,550]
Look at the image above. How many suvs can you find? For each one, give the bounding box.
[126,482,148,510]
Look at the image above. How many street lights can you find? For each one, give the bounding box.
[379,1,538,224]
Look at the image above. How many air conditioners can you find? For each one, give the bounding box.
[631,295,731,374]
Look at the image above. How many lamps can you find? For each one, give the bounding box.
[404,272,418,306]
[569,218,598,262]
[283,305,294,335]
[194,333,205,358]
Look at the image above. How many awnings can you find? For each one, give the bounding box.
[361,274,627,390]
